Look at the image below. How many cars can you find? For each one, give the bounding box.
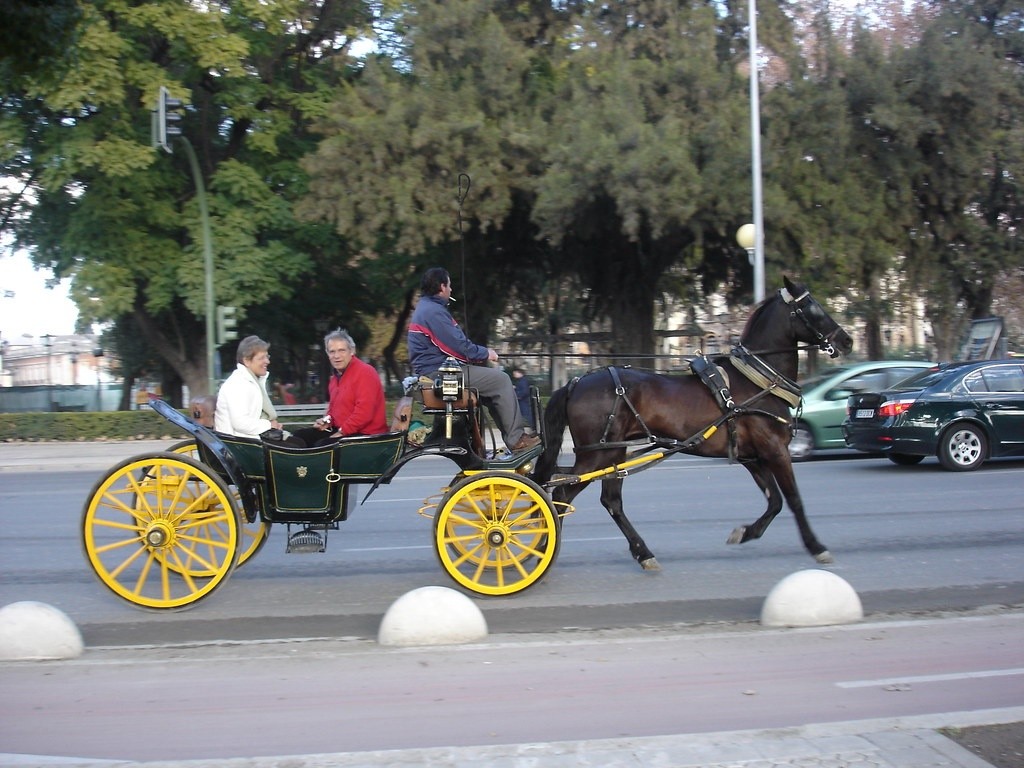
[840,357,1024,471]
[790,359,944,462]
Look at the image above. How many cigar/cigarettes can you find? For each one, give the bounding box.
[449,296,456,302]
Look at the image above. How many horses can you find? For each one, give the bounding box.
[533,274,854,571]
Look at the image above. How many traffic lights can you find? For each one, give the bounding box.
[156,85,187,157]
[216,303,241,345]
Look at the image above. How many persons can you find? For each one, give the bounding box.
[407,267,541,455]
[212,336,306,453]
[294,327,388,448]
[511,367,533,428]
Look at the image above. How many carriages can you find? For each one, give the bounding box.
[79,273,856,614]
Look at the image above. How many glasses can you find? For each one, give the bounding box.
[249,354,270,361]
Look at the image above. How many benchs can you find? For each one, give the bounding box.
[190,394,264,475]
[338,396,414,477]
[418,376,478,409]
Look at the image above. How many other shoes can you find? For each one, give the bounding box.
[512,433,542,453]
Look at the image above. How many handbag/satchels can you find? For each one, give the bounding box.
[260,428,283,442]
